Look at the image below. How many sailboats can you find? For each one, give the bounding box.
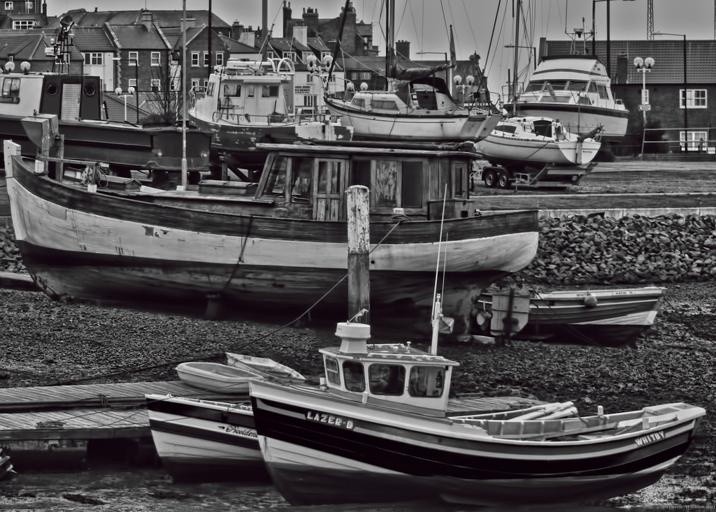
[474,1,605,173]
[322,1,506,144]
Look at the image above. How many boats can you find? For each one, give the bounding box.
[502,1,632,138]
[187,2,324,141]
[1,139,541,326]
[248,183,709,509]
[143,390,580,482]
[471,285,670,341]
[223,351,307,386]
[174,360,266,393]
